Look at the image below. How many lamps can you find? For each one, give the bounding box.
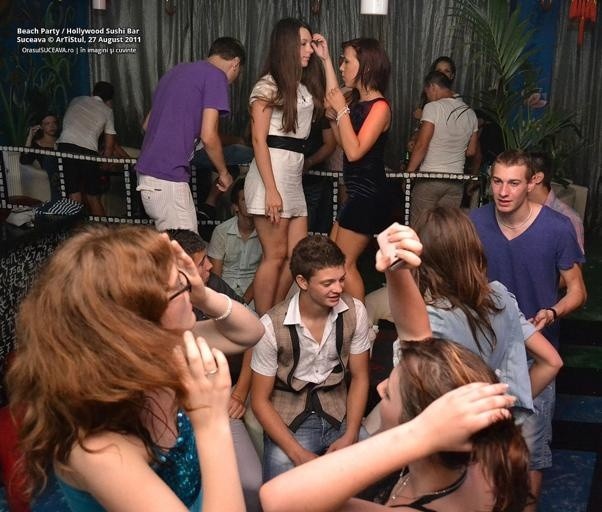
[92,0,106,10]
[360,0,389,15]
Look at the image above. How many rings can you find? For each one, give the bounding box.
[205,369,219,376]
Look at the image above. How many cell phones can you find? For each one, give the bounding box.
[376,221,406,273]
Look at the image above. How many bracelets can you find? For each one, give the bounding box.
[206,292,232,321]
[546,307,557,327]
[232,393,248,409]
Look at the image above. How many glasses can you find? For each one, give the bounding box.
[168,269,192,301]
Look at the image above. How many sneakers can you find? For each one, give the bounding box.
[197,202,216,220]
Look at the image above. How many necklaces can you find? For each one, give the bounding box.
[392,476,466,500]
[499,202,533,229]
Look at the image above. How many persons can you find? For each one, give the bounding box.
[526,152,586,290]
[258,226,538,512]
[392,204,564,426]
[19,114,59,168]
[58,81,118,217]
[165,229,263,512]
[3,223,267,512]
[468,150,587,512]
[135,18,505,318]
[249,235,372,484]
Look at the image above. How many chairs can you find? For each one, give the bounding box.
[19,160,51,202]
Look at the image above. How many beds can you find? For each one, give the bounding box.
[89,146,141,173]
